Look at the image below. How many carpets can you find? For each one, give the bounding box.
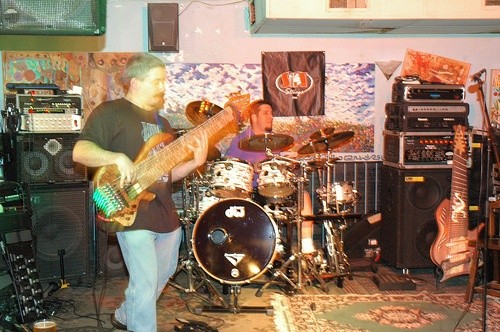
[275,290,499,332]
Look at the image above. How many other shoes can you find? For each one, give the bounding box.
[110,313,127,330]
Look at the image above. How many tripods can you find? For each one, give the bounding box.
[162,173,237,314]
[255,169,329,296]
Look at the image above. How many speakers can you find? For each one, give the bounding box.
[0,131,87,186]
[379,162,471,273]
[20,181,97,284]
[148,3,180,53]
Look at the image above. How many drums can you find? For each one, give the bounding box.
[191,196,281,285]
[256,157,300,199]
[318,179,357,208]
[206,157,254,199]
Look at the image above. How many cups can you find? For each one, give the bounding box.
[33,318,58,332]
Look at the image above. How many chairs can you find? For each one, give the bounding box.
[464,201,500,304]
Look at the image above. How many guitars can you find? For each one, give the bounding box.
[429,126,484,283]
[92,90,252,229]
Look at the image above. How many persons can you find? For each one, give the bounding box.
[223,98,289,270]
[72,52,209,332]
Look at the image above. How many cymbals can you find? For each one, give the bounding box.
[296,130,356,155]
[309,127,335,140]
[184,99,224,127]
[237,132,295,153]
